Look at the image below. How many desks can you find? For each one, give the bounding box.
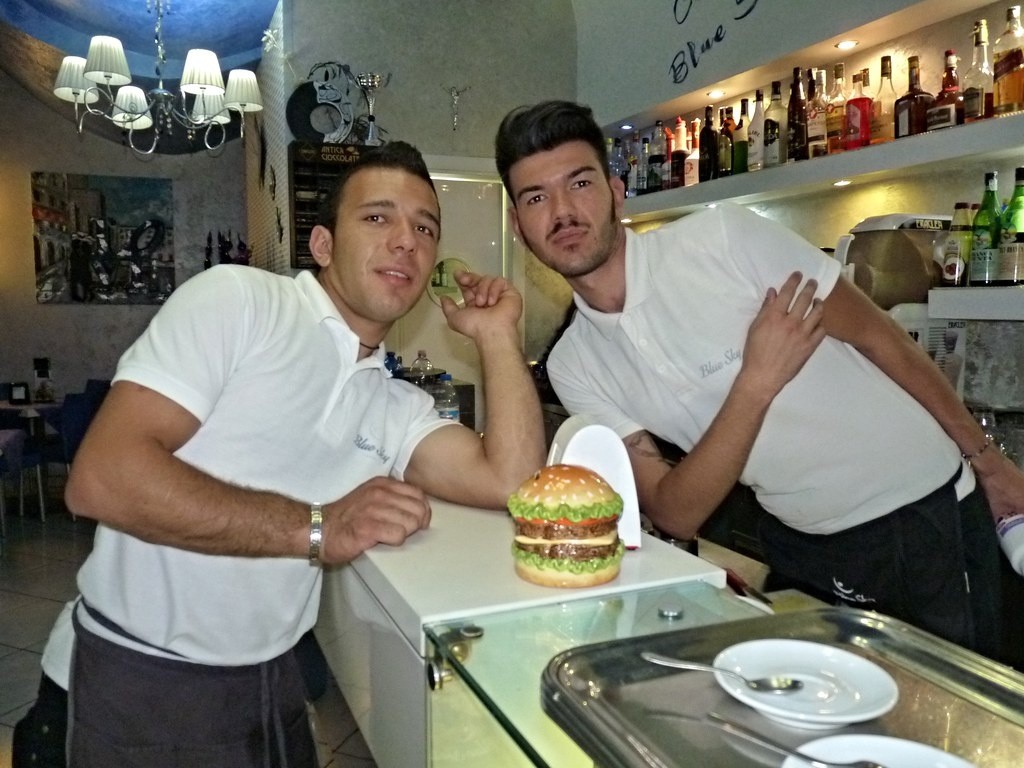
[0,397,66,438]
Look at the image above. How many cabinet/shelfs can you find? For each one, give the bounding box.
[620,114,1024,323]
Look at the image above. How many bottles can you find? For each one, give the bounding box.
[431,374,461,423]
[409,349,434,394]
[604,3,1024,287]
[384,351,397,371]
[394,356,404,379]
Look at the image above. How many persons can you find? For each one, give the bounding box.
[494,101,1024,669]
[66,141,546,768]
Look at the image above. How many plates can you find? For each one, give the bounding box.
[782,731,978,768]
[713,638,899,730]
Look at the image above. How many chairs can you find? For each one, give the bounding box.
[0,428,28,541]
[84,377,113,395]
[38,397,93,529]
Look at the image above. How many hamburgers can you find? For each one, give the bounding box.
[507,464,624,588]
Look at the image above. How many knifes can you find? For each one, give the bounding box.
[727,569,772,604]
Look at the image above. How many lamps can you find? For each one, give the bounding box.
[54,0,266,154]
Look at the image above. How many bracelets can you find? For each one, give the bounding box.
[962,435,993,458]
[309,503,322,564]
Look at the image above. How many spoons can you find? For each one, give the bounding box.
[705,710,886,768]
[639,650,804,695]
